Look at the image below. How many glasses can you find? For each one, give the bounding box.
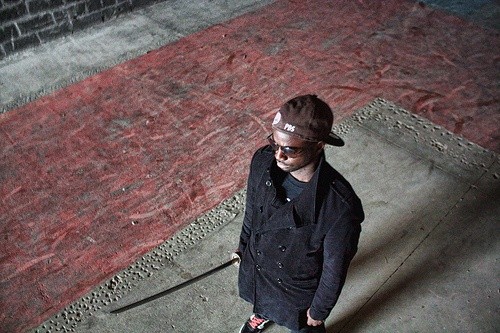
[268,133,319,154]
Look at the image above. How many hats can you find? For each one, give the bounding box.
[271,95,344,146]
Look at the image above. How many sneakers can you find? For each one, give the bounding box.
[241,314,273,333]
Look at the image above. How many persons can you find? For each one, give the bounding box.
[236,94,365,333]
